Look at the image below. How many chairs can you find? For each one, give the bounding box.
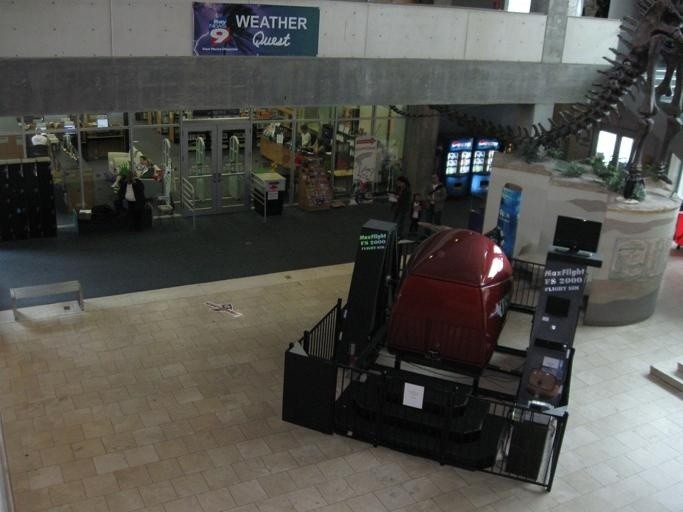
[107,145,138,175]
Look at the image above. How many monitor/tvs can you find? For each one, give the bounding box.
[64,120,75,125]
[552,215,603,257]
[97,115,108,118]
[63,125,75,134]
[97,118,109,128]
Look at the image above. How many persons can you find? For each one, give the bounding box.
[116,173,145,233]
[111,156,147,188]
[424,173,447,225]
[392,176,412,237]
[114,160,152,194]
[409,193,427,232]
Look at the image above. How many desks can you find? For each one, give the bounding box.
[22,123,129,159]
[327,169,354,199]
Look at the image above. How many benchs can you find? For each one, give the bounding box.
[9,278,87,321]
[132,151,163,199]
